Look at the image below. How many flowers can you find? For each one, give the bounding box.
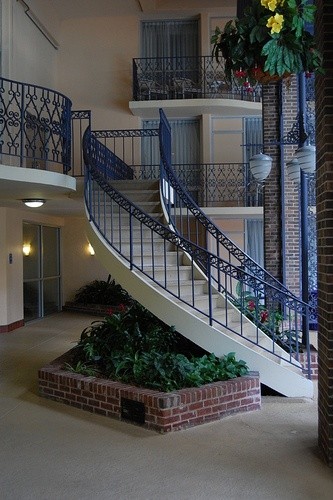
[211,0,327,93]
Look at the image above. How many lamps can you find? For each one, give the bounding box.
[250,145,273,181]
[295,139,316,174]
[88,242,95,256]
[21,199,46,208]
[286,155,301,185]
[23,241,31,257]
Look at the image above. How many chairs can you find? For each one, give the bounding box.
[137,79,169,100]
[172,78,201,99]
[207,80,227,99]
[238,180,264,207]
